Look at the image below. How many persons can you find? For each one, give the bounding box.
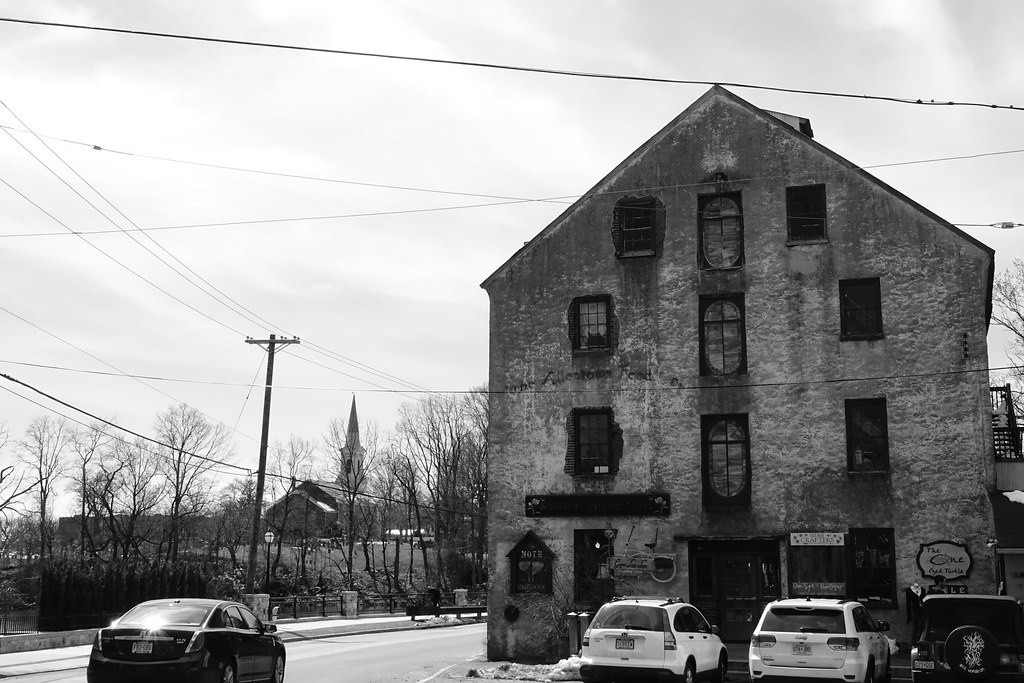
[927,576,947,594]
[906,583,927,647]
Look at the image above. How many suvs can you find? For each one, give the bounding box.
[577,595,729,683]
[910,592,1024,683]
[748,595,892,683]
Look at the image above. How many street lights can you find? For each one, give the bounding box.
[265,526,275,595]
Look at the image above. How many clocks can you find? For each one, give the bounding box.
[649,556,677,584]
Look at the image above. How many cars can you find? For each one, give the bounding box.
[87,598,286,683]
